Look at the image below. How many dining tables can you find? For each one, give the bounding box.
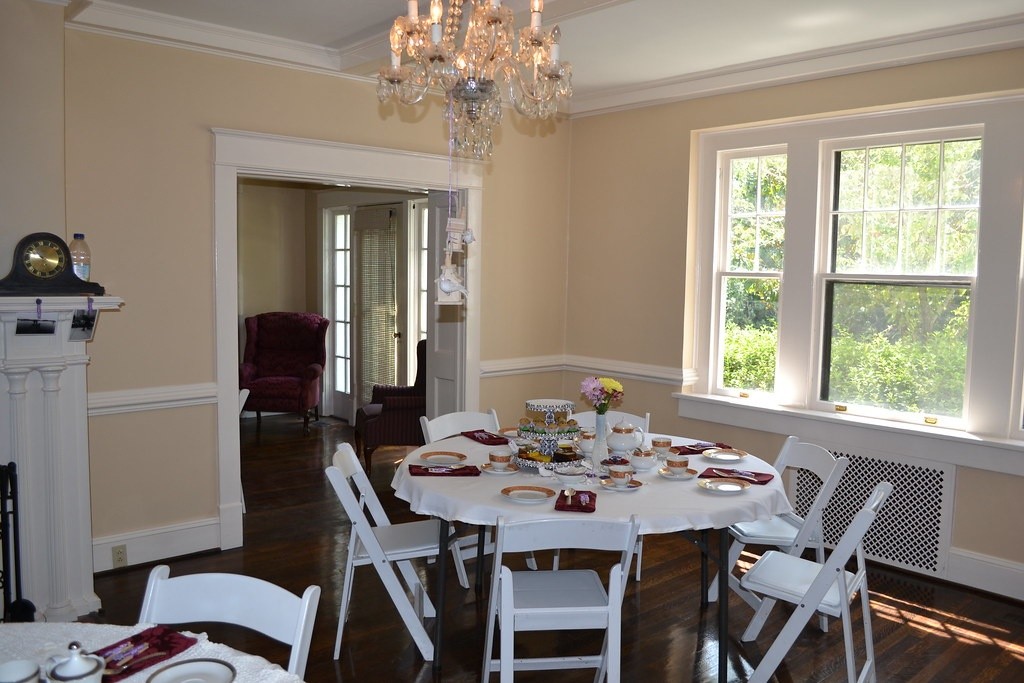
[0,622,307,683]
[391,426,794,683]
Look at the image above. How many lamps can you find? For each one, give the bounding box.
[376,0,574,161]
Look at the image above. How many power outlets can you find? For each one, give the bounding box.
[112,544,127,568]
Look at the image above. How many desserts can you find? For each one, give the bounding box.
[517,416,581,463]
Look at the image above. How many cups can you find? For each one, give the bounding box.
[0,659,39,683]
[488,450,513,471]
[609,437,689,488]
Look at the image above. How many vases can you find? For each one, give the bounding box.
[592,411,609,468]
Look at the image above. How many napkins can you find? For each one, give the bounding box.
[554,489,597,513]
[89,625,198,683]
[408,464,480,477]
[672,442,732,455]
[697,467,774,485]
[460,429,509,446]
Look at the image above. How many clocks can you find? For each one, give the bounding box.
[0,232,105,296]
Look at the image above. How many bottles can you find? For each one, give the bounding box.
[44,640,106,683]
[69,234,90,282]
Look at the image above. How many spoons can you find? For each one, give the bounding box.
[564,488,577,506]
[417,463,465,469]
[685,445,722,450]
[102,651,166,675]
[713,469,757,481]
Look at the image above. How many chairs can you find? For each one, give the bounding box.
[239,312,330,438]
[139,564,322,681]
[325,408,892,683]
[354,340,426,475]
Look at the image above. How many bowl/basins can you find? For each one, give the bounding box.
[554,465,587,485]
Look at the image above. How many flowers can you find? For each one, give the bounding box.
[578,376,625,414]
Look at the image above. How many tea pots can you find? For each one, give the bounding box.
[604,417,658,471]
[572,432,596,457]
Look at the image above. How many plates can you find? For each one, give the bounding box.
[481,463,519,474]
[697,478,751,493]
[420,452,467,464]
[650,448,698,480]
[145,657,236,683]
[600,479,642,491]
[501,486,556,503]
[702,449,749,461]
[499,427,518,437]
